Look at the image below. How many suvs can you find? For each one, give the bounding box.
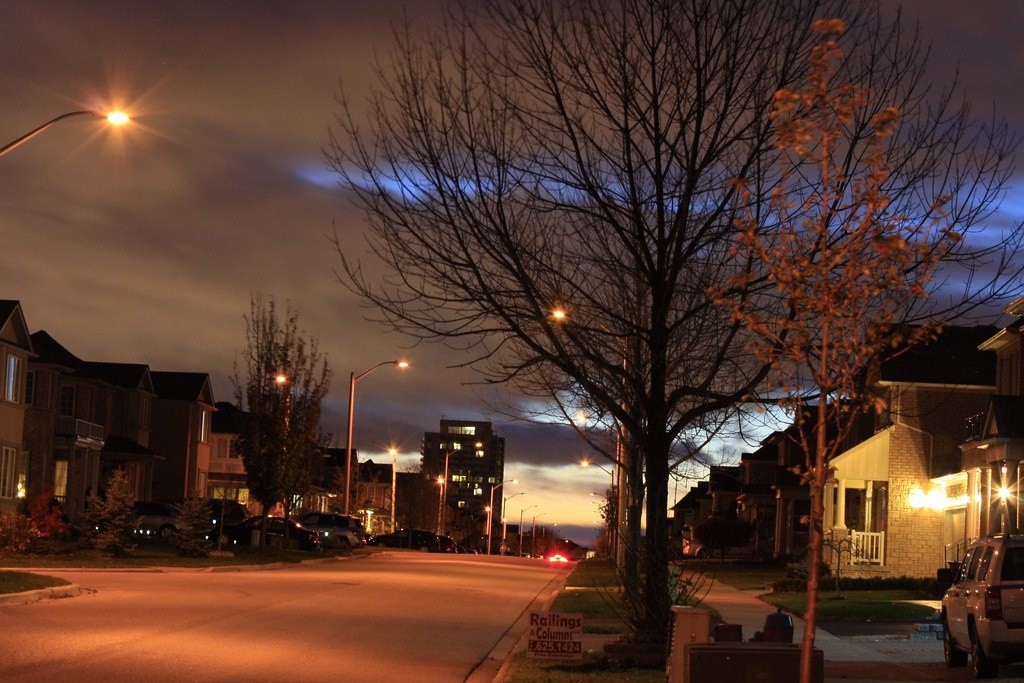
[132,501,184,538]
[942,533,1024,680]
[299,512,367,549]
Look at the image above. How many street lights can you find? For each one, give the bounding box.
[532,513,548,559]
[488,480,513,554]
[502,493,526,556]
[486,507,490,528]
[444,442,482,514]
[389,448,397,533]
[341,360,408,511]
[554,310,628,580]
[0,105,129,157]
[519,505,539,557]
[581,462,614,490]
[437,478,444,535]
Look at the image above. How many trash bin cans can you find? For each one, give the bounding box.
[664,605,711,683]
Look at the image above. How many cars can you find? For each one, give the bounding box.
[437,535,458,553]
[532,552,545,559]
[457,545,467,553]
[189,497,250,527]
[210,515,321,551]
[681,536,750,557]
[368,529,441,552]
[496,544,512,553]
[506,550,519,556]
